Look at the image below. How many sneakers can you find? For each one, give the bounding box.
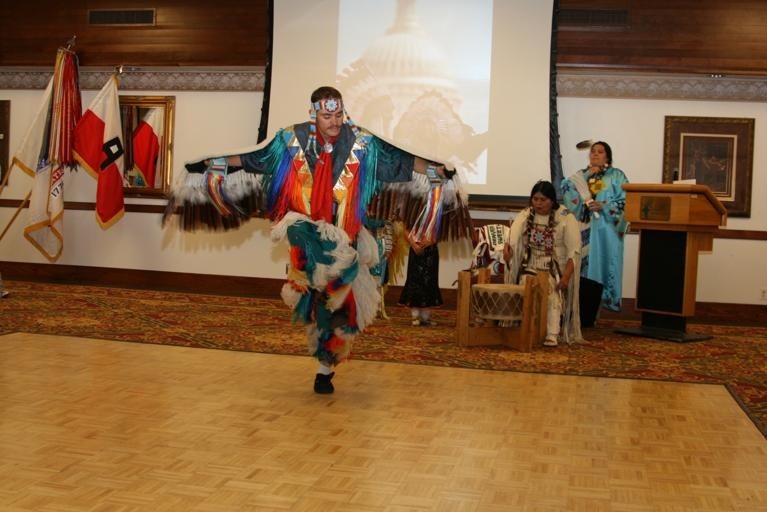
[543,334,558,346]
[313,371,335,394]
[412,316,439,327]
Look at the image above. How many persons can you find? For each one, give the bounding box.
[183,84,452,395]
[502,178,584,348]
[559,140,630,339]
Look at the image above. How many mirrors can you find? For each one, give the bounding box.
[119,95,176,198]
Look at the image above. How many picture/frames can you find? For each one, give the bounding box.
[662,115,755,218]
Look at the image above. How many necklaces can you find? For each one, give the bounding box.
[318,128,334,155]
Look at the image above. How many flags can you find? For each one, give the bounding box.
[126,102,163,190]
[72,75,126,230]
[13,45,84,266]
